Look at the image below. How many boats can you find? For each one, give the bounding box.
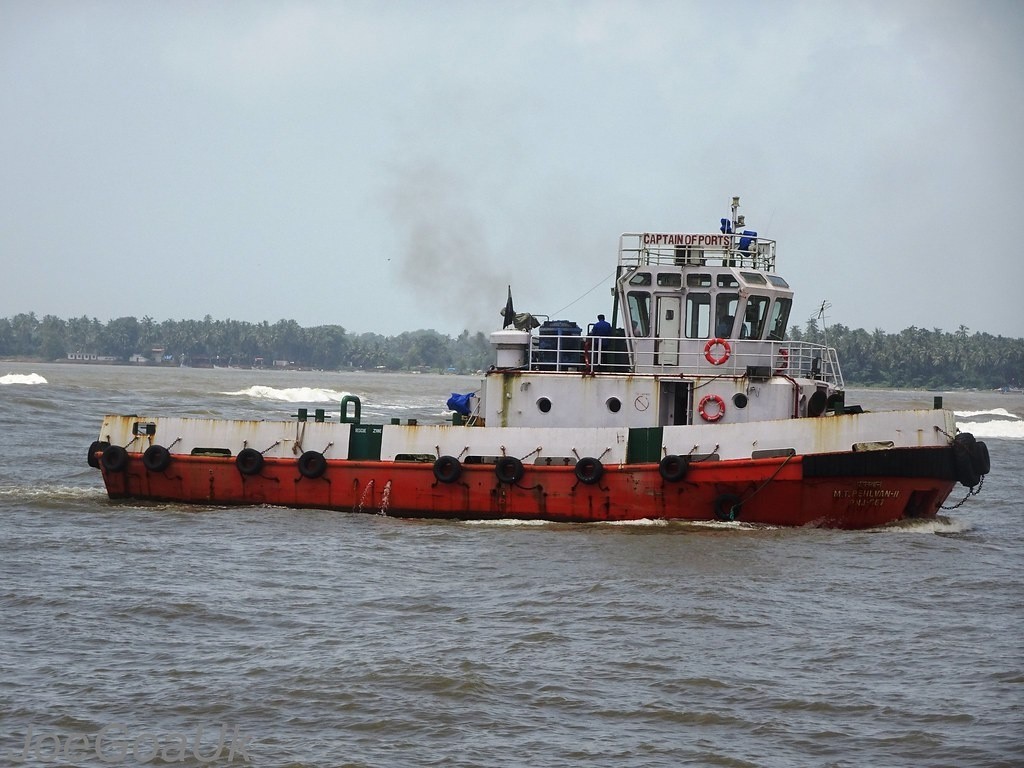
[86,193,992,532]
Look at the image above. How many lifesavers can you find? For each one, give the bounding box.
[102,444,129,472]
[975,441,990,477]
[143,445,169,472]
[713,492,743,519]
[495,456,523,483]
[774,349,787,377]
[699,395,725,422]
[237,448,265,475]
[705,338,731,364]
[296,450,327,477]
[574,457,603,484]
[433,456,462,483]
[88,440,100,468]
[658,454,687,483]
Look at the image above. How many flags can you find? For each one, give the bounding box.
[502,285,513,329]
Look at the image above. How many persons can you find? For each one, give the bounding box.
[631,320,641,337]
[590,312,613,372]
